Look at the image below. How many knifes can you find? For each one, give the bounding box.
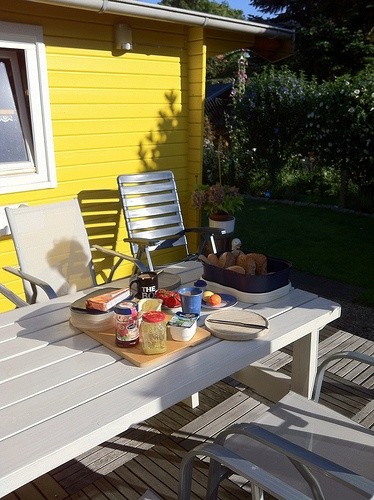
[152,269,164,279]
[207,319,268,330]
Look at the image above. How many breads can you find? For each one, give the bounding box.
[207,250,268,276]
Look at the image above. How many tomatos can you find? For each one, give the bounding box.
[156,288,181,308]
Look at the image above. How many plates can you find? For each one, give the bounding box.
[201,293,238,310]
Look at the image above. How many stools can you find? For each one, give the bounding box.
[199,232,239,254]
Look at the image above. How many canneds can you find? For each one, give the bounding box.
[114,300,139,348]
[139,311,167,354]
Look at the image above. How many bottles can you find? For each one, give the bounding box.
[138,311,167,355]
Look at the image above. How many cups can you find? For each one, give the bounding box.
[178,286,203,320]
[129,271,159,300]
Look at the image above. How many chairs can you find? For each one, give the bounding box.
[2,199,147,305]
[205,352,374,500]
[118,171,226,273]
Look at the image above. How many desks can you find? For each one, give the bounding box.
[0,260,340,499]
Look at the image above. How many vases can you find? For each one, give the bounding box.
[208,217,235,234]
[209,214,228,221]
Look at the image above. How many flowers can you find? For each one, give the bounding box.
[191,182,244,216]
[215,47,250,109]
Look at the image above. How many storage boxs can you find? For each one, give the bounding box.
[200,252,292,303]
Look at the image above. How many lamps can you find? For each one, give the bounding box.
[114,24,132,50]
